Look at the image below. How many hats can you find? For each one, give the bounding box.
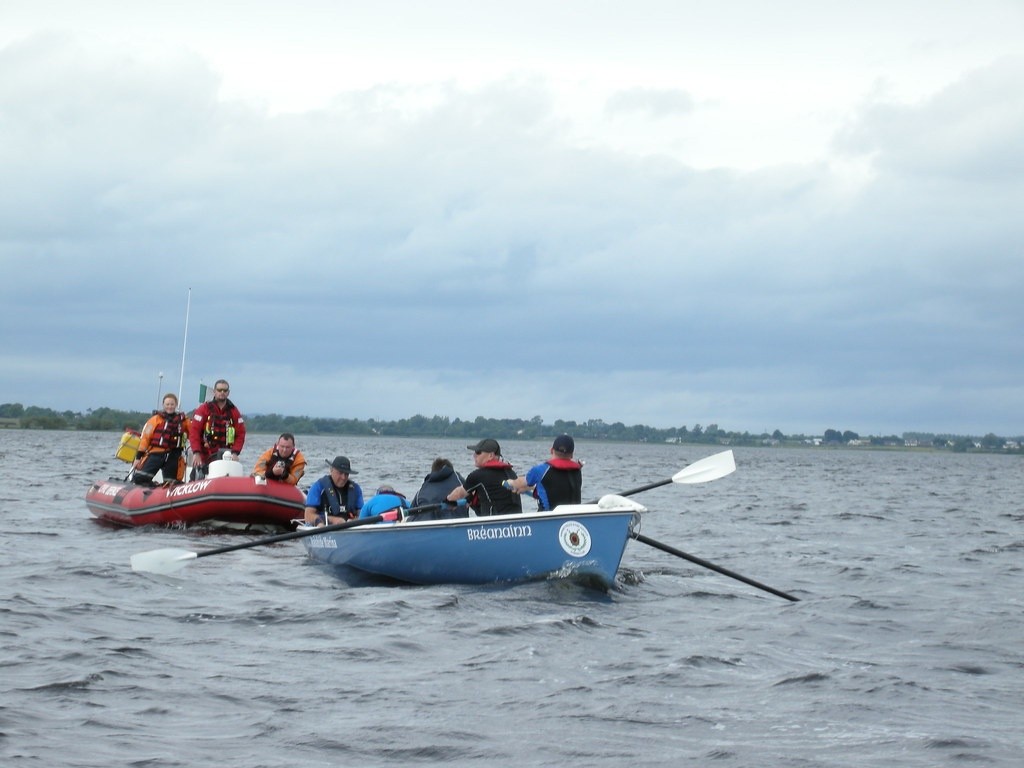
[466,440,500,456]
[553,435,574,455]
[325,456,359,475]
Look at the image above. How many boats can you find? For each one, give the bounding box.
[85,480,307,529]
[285,492,644,593]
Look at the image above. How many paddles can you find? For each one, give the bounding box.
[127,498,468,577]
[581,447,736,505]
[500,480,798,601]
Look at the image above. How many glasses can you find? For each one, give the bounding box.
[215,388,229,393]
[475,449,481,455]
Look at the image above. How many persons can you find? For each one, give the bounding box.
[252,433,307,485]
[131,393,191,486]
[304,456,364,528]
[506,436,581,514]
[189,380,246,483]
[358,485,411,524]
[444,439,523,516]
[406,458,469,523]
